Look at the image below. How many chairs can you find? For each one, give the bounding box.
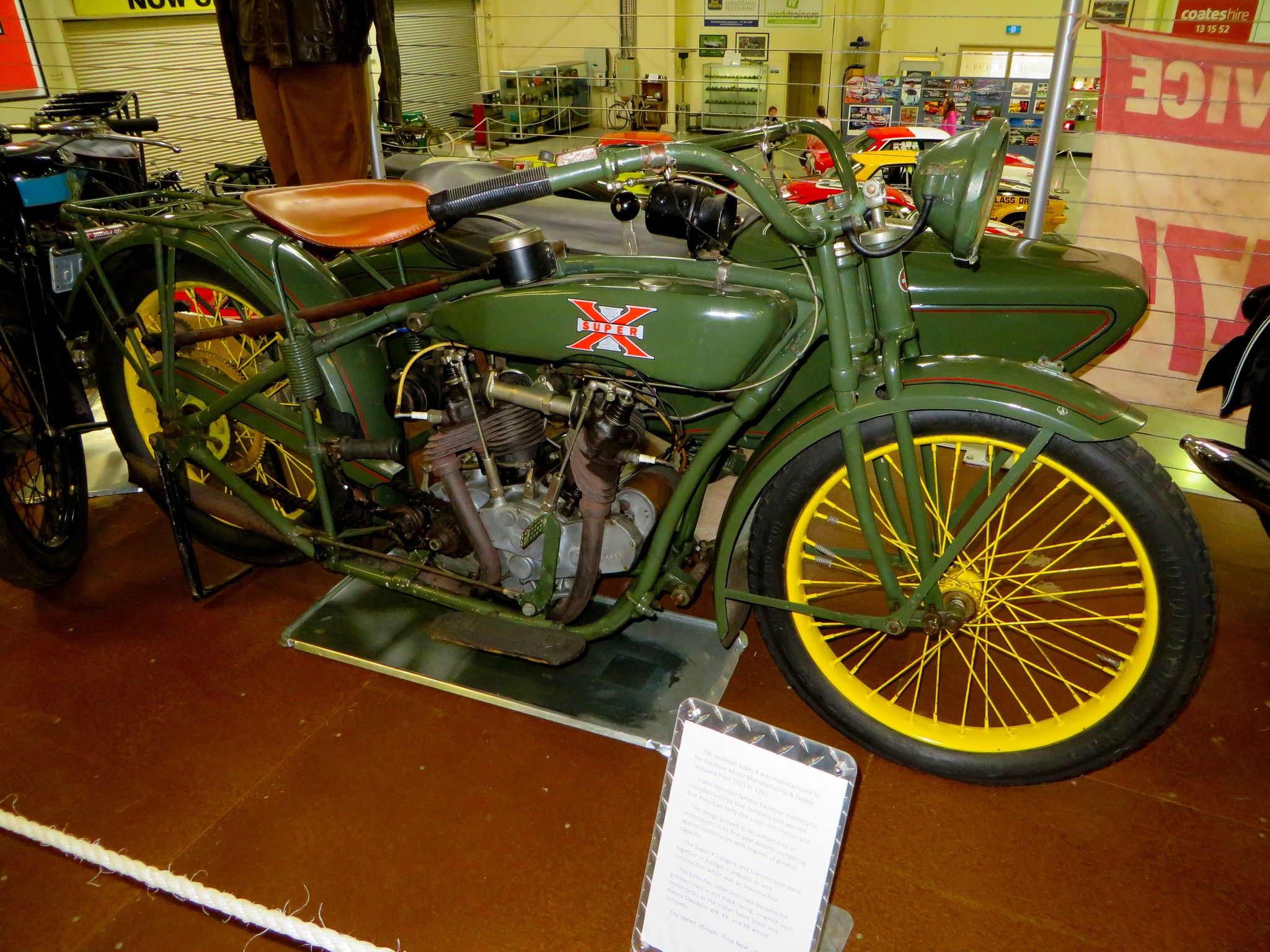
[240,179,437,248]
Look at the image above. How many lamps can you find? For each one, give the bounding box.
[849,36,870,67]
[671,49,697,103]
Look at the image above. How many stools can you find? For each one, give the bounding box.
[688,113,701,132]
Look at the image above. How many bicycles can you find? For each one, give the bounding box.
[608,93,662,133]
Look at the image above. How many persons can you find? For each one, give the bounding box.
[941,97,958,137]
[762,106,778,171]
[902,80,920,106]
[799,105,832,170]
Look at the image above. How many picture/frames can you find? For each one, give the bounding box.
[1085,0,1135,29]
[0,0,49,104]
[735,32,769,61]
[699,34,727,57]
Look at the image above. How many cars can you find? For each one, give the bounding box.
[774,177,1025,237]
[820,148,1069,235]
[803,126,1036,178]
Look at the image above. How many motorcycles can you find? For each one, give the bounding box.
[0,116,1221,788]
[377,114,456,158]
[1176,281,1270,539]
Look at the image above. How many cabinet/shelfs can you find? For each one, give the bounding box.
[499,61,590,140]
[702,63,769,131]
[637,78,668,130]
